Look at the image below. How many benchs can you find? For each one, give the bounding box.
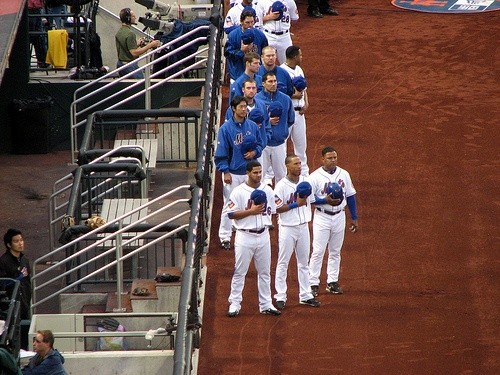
[94,197,149,281]
[110,139,159,197]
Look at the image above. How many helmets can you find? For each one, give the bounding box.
[327,183,344,206]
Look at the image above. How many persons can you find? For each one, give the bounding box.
[307,0,339,18]
[225,161,282,316]
[225,79,269,174]
[224,0,299,84]
[273,154,320,309]
[214,96,264,250]
[116,7,161,78]
[256,46,294,99]
[280,46,310,177]
[230,53,260,105]
[26,0,51,69]
[0,228,32,321]
[308,147,359,296]
[254,72,295,185]
[20,329,68,375]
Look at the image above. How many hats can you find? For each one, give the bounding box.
[293,181,312,199]
[251,190,267,212]
[243,6,256,18]
[249,109,264,123]
[268,101,282,117]
[240,135,258,157]
[293,76,306,91]
[271,1,284,21]
[241,29,255,45]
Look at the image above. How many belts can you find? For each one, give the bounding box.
[241,227,265,234]
[293,107,303,111]
[317,207,341,215]
[265,29,288,35]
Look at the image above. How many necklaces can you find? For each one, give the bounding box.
[285,62,294,69]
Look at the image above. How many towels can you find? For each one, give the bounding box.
[45,28,68,69]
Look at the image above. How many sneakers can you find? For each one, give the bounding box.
[226,308,240,317]
[276,301,286,310]
[221,242,231,249]
[260,307,282,317]
[299,298,321,307]
[326,283,343,294]
[310,286,320,297]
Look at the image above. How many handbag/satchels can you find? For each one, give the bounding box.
[98,323,124,351]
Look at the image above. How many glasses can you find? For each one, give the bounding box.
[34,339,40,343]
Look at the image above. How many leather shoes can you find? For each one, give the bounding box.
[320,7,339,16]
[307,8,323,17]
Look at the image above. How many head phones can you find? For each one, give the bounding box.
[124,8,131,22]
